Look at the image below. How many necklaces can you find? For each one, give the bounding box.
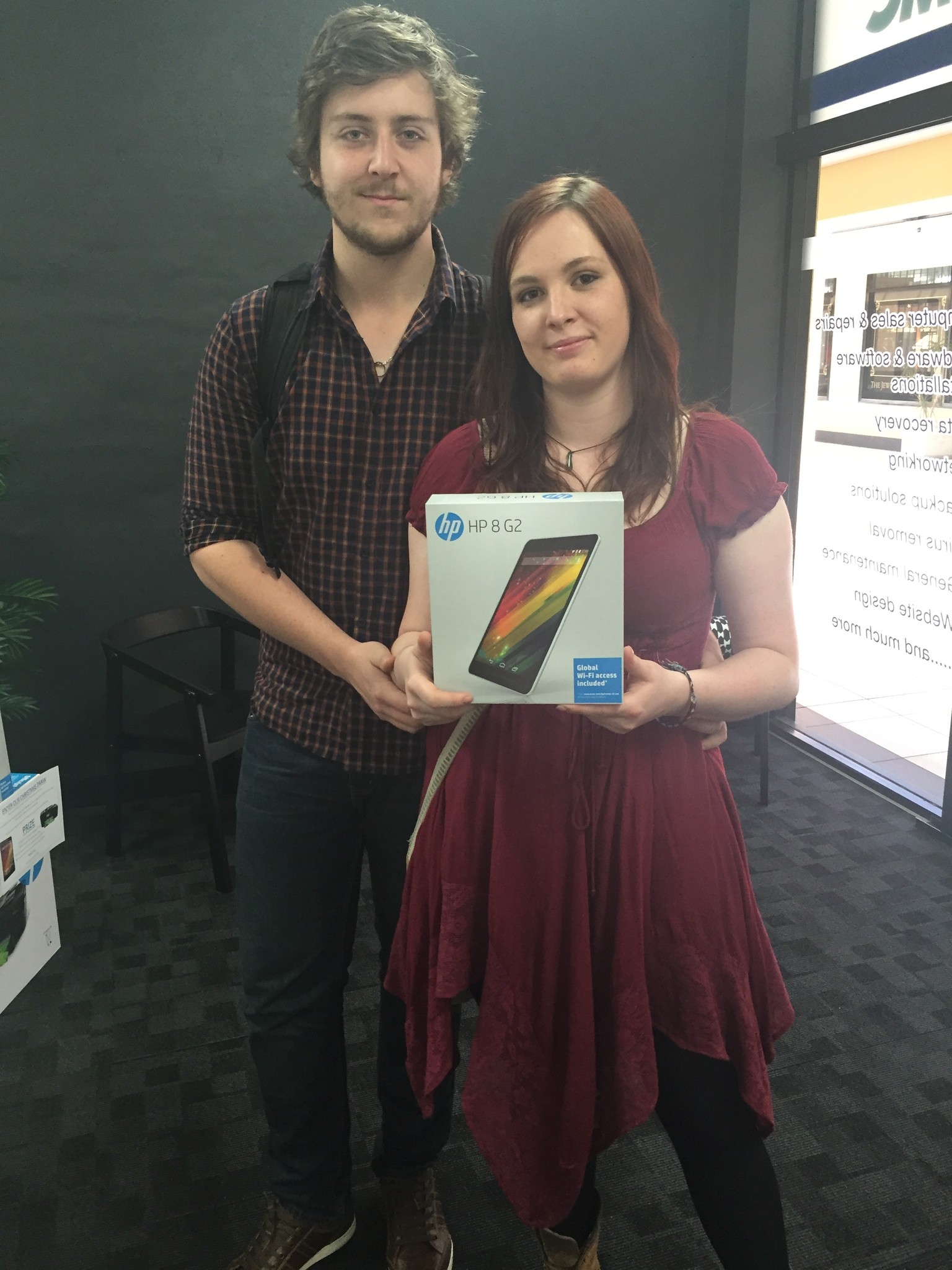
[373,356,393,377]
[544,428,625,473]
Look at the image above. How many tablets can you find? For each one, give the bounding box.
[467,535,603,697]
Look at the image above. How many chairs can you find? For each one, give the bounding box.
[98,603,261,894]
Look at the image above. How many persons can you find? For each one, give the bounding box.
[388,176,799,1269]
[181,5,502,1269]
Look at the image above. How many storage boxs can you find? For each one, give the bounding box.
[0,772,62,1014]
[423,490,626,705]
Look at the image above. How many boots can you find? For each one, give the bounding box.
[528,1184,606,1270]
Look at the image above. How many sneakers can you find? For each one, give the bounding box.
[377,1161,457,1270]
[218,1192,356,1270]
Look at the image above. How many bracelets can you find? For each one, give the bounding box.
[656,661,696,731]
